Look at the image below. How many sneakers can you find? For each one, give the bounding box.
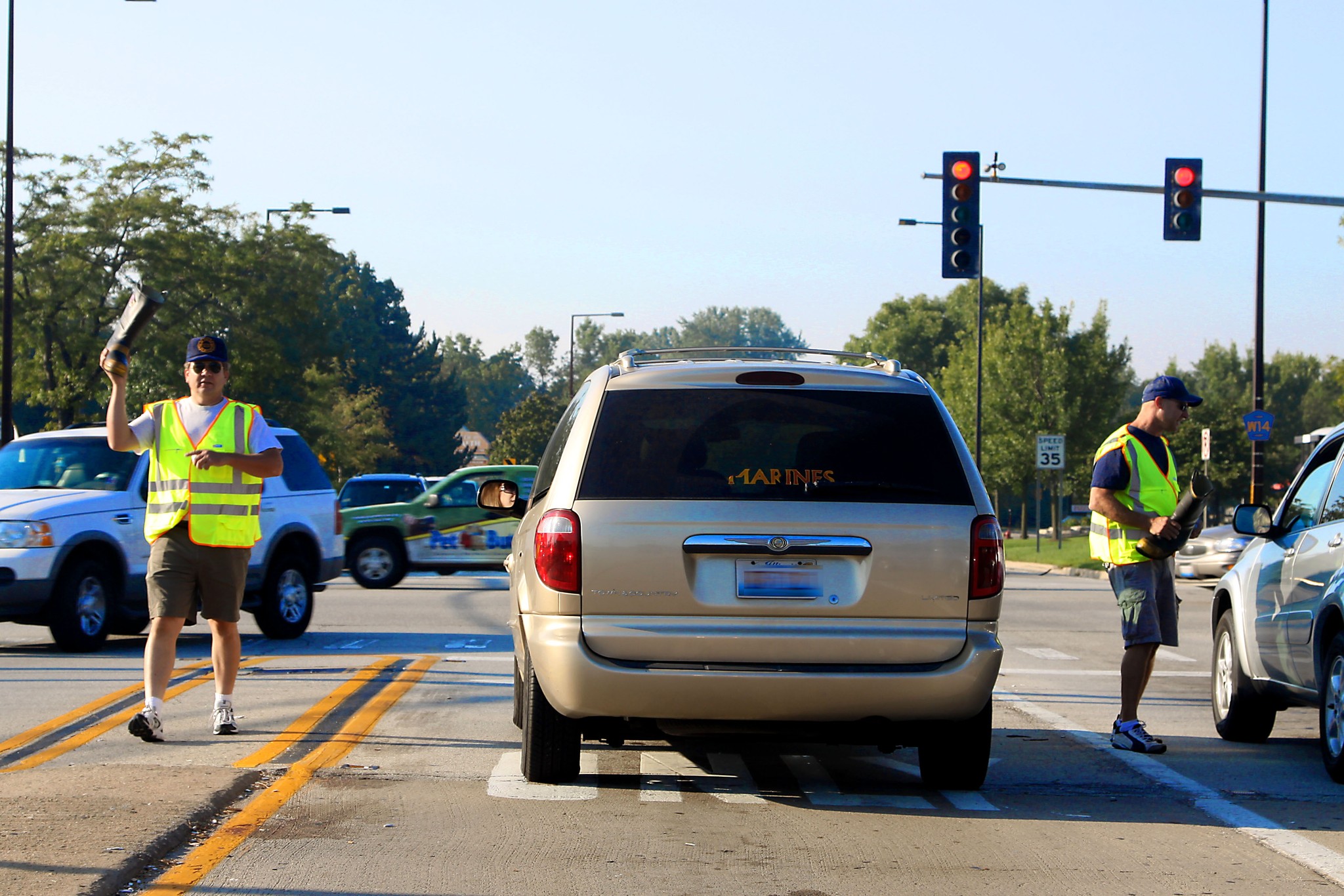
[1110,714,1164,744]
[126,701,165,743]
[208,700,239,736]
[1111,720,1167,755]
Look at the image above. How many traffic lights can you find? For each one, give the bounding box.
[940,152,982,282]
[1164,155,1206,244]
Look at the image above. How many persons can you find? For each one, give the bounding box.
[1088,377,1203,754]
[481,482,516,507]
[99,336,284,742]
[1254,508,1270,532]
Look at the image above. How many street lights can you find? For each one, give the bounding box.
[897,216,989,473]
[264,201,354,299]
[564,310,632,402]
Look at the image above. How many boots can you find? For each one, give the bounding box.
[101,283,170,378]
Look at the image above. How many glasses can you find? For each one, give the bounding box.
[499,482,517,495]
[187,360,224,374]
[1169,399,1189,411]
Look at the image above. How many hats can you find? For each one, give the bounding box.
[1142,376,1205,408]
[185,334,228,362]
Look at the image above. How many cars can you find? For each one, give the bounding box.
[1172,524,1252,579]
[330,473,481,525]
[1205,419,1344,787]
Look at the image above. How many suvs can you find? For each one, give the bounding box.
[338,463,541,589]
[474,344,1011,794]
[0,418,345,657]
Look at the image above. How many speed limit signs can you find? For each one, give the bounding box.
[1036,432,1070,473]
